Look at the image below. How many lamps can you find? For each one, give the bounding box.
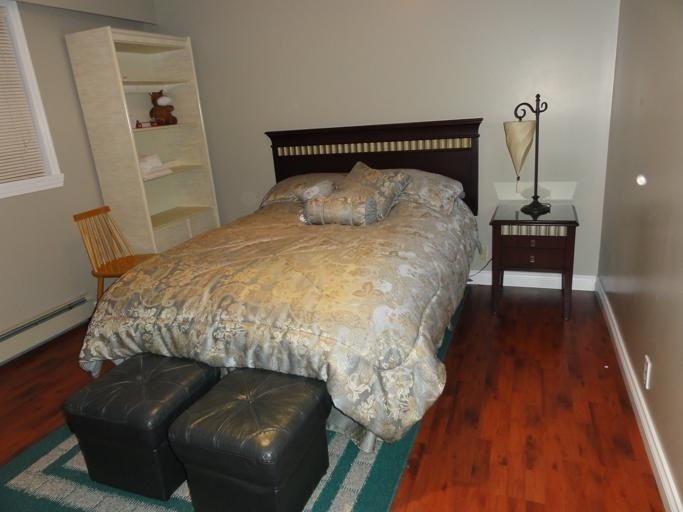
[504,94,551,217]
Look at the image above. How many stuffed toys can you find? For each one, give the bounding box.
[148,90,177,126]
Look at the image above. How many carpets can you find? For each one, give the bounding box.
[2,271,469,512]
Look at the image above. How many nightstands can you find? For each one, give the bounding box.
[490,202,578,320]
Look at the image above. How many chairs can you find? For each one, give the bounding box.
[73,206,161,302]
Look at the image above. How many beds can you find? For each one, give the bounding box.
[123,118,482,443]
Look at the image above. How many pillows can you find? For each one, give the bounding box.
[259,160,465,226]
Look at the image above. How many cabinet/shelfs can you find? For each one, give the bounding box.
[62,25,222,254]
